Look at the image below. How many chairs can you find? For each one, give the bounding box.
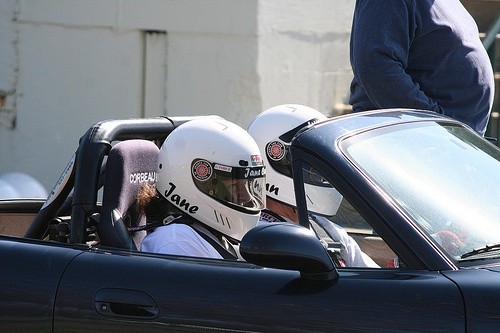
[96,137,162,252]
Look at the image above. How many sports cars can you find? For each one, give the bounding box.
[1,107,500,333]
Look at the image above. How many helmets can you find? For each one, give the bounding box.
[247,104,344,217]
[155,116,267,242]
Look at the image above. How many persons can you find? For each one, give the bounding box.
[348,0,495,138]
[135,116,267,262]
[249,104,380,269]
